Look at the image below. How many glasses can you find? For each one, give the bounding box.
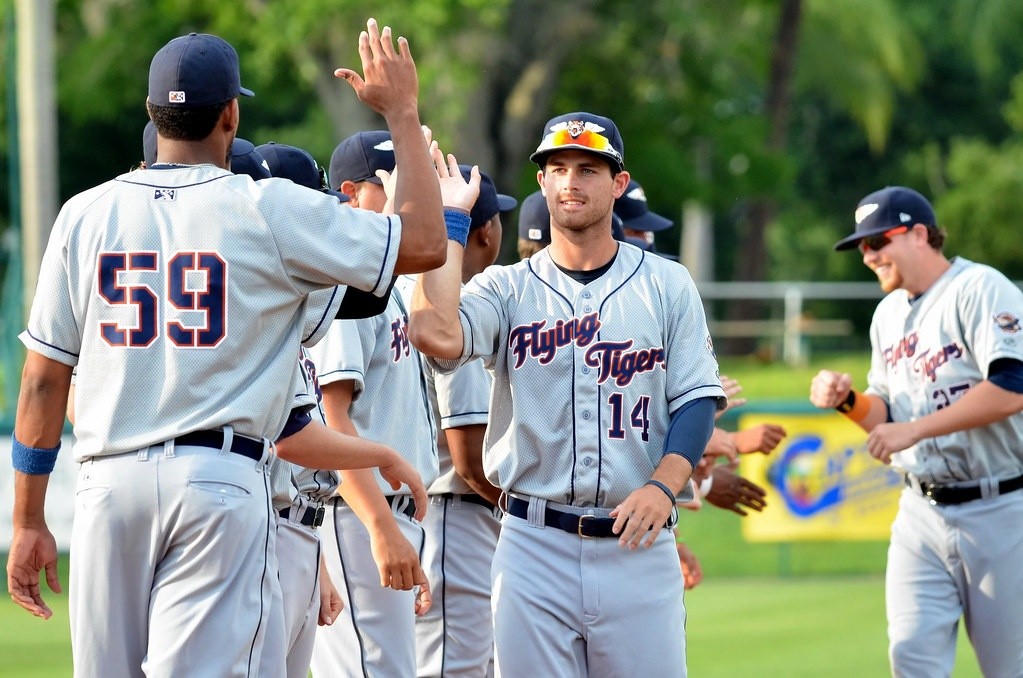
[858,227,908,255]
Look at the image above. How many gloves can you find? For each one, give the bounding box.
[375,127,439,215]
[434,150,481,213]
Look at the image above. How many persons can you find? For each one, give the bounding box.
[6,18,448,678]
[809,187,1023,678]
[142,124,786,678]
[407,112,728,678]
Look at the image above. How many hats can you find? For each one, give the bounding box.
[143,121,157,167]
[529,113,625,173]
[328,131,396,192]
[227,138,271,181]
[517,191,553,243]
[148,33,255,109]
[255,141,350,203]
[612,216,647,250]
[834,187,936,252]
[614,179,674,231]
[445,164,517,228]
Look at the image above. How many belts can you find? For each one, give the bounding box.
[505,497,674,539]
[448,494,496,513]
[388,497,416,517]
[155,429,271,467]
[279,502,325,530]
[904,475,1021,507]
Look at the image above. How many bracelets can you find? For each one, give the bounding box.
[838,388,872,423]
[699,474,712,497]
[645,480,676,506]
[444,210,471,248]
[11,430,62,475]
